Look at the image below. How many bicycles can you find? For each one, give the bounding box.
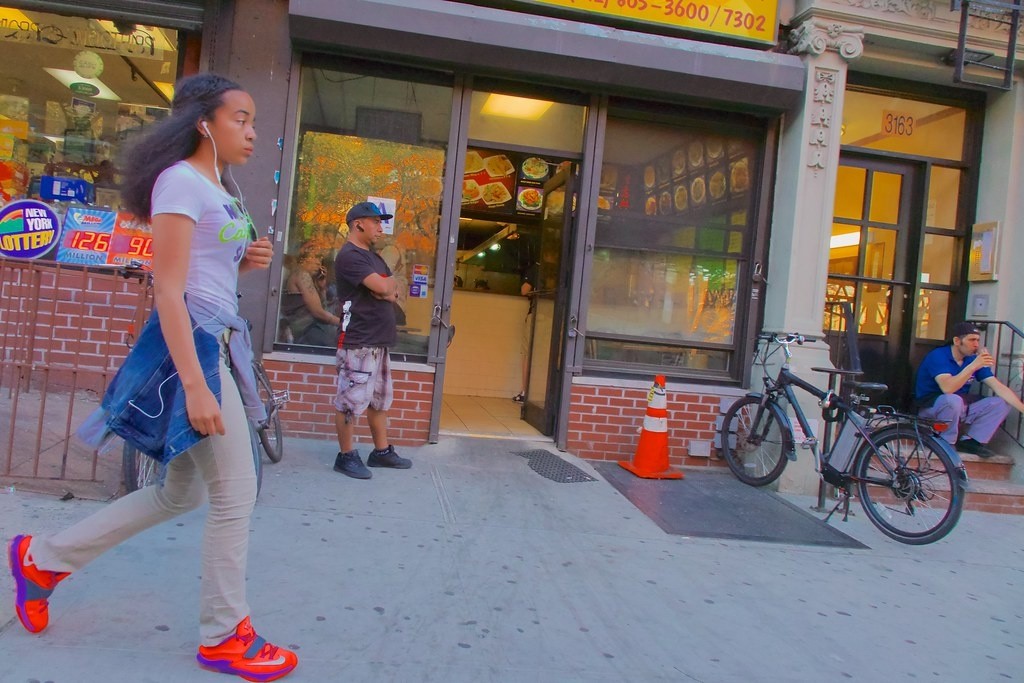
[122,258,286,494]
[720,328,969,546]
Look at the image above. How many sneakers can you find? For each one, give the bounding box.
[511,393,526,404]
[6,535,72,637]
[367,446,413,470]
[333,448,373,480]
[192,618,299,682]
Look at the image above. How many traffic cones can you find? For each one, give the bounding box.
[617,374,685,480]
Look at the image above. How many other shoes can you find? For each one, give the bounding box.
[955,435,996,460]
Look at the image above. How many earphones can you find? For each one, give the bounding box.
[202,121,210,138]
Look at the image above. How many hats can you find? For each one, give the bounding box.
[943,322,982,345]
[345,200,394,227]
[474,279,492,289]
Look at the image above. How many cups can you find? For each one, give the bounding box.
[976,347,992,367]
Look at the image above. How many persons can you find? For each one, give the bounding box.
[475,280,491,290]
[392,291,408,333]
[511,241,560,404]
[281,240,342,349]
[92,160,121,190]
[453,275,464,287]
[913,321,1024,458]
[4,70,299,683]
[333,200,413,480]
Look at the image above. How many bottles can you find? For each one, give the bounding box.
[27,123,36,144]
[0,192,110,214]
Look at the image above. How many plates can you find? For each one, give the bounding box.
[642,133,748,217]
[461,178,481,203]
[544,159,615,218]
[522,157,548,178]
[480,182,512,205]
[463,150,485,174]
[483,154,515,177]
[518,189,542,210]
